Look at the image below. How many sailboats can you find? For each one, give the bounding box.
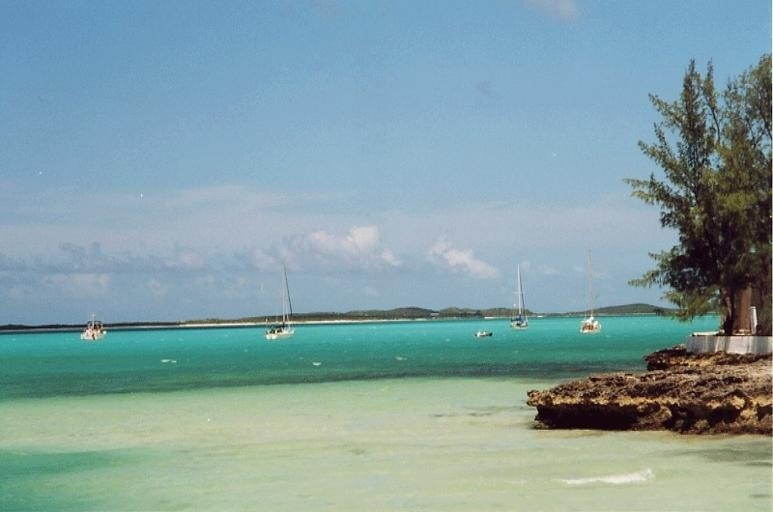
[263,262,294,342]
[578,247,602,335]
[510,263,528,331]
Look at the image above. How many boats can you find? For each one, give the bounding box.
[475,330,493,338]
[80,311,107,341]
